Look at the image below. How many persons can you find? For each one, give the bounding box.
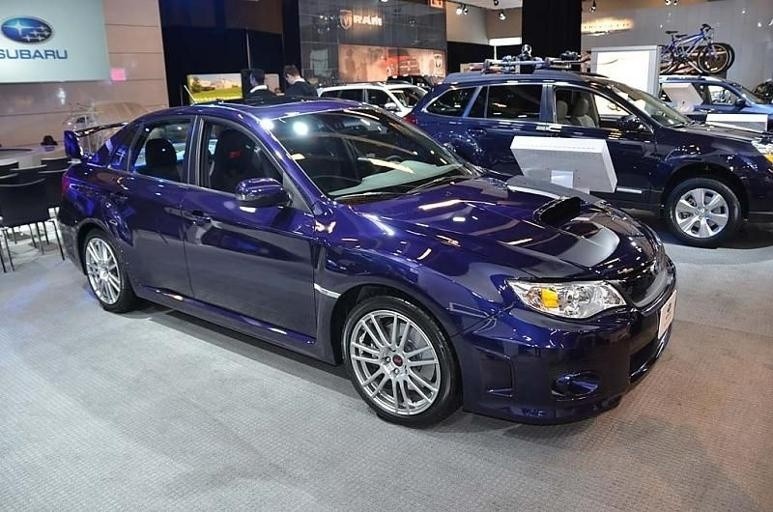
[281,64,318,97]
[245,67,276,97]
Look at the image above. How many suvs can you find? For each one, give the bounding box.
[401,44,773,250]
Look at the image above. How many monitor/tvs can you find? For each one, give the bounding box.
[183,72,244,105]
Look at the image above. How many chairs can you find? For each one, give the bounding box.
[1,156,72,273]
[137,129,332,195]
[554,98,596,129]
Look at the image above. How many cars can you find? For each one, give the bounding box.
[55,94,679,430]
[313,74,451,119]
[657,76,772,126]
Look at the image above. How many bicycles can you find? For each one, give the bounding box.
[657,24,735,75]
